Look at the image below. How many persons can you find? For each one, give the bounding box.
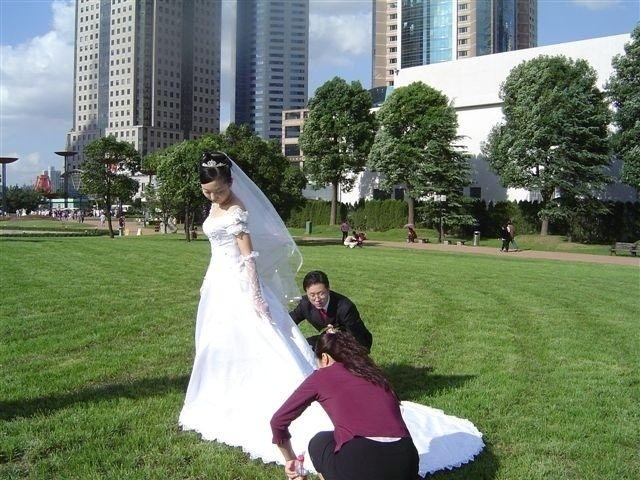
[506,220,519,252]
[291,270,373,357]
[15,206,115,226]
[118,214,126,227]
[176,149,305,480]
[270,326,422,480]
[172,216,178,226]
[407,228,417,243]
[501,224,513,252]
[341,219,366,249]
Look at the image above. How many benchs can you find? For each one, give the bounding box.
[609,240,640,256]
[405,236,427,243]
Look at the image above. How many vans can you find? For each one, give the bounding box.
[111,205,133,217]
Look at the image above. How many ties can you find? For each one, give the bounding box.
[319,308,326,320]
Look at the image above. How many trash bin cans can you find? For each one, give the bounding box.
[118,227,124,236]
[474,231,480,246]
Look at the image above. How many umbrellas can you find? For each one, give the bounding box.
[403,223,414,229]
